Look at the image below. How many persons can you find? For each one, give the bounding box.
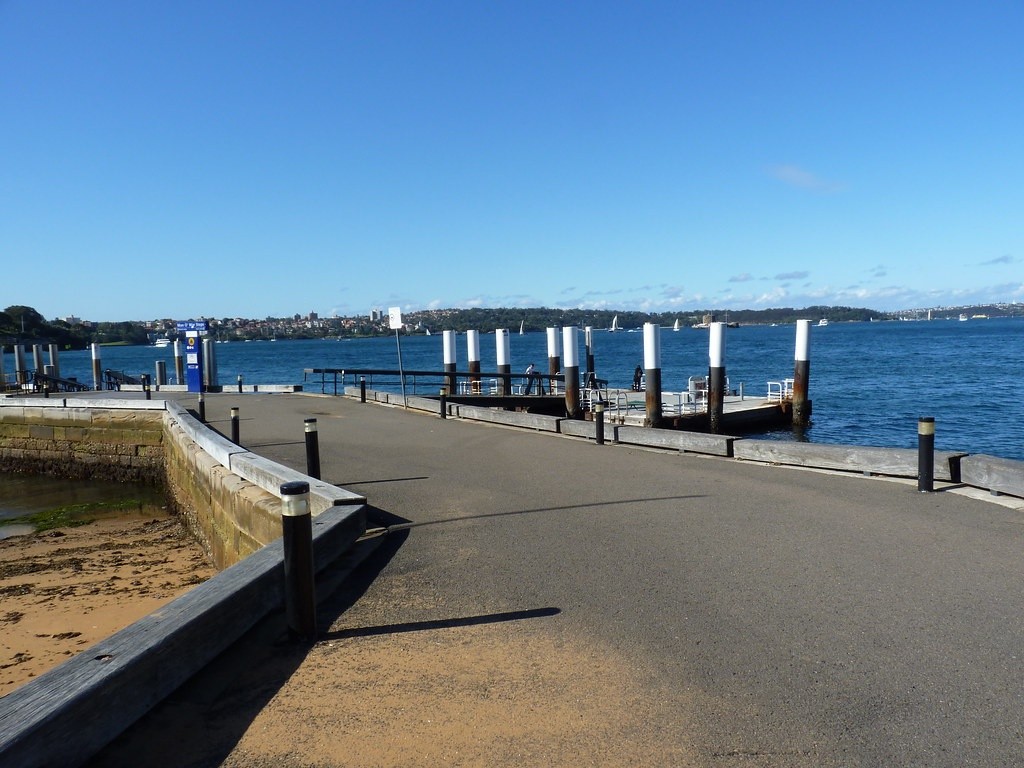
[634,365,643,392]
[525,364,534,395]
